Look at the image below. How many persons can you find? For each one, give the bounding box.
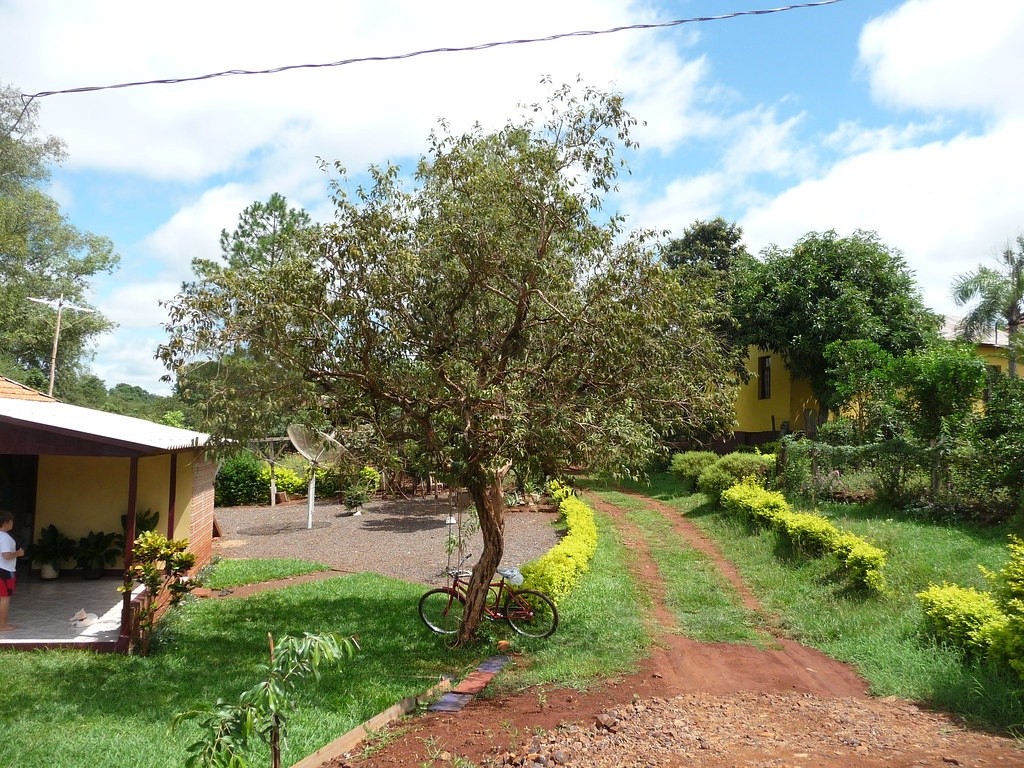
[0,510,24,631]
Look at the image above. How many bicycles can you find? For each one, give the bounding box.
[418,554,559,639]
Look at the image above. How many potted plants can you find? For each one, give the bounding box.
[26,523,77,581]
[114,508,160,578]
[70,530,122,579]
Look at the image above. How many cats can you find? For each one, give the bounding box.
[68,608,99,628]
